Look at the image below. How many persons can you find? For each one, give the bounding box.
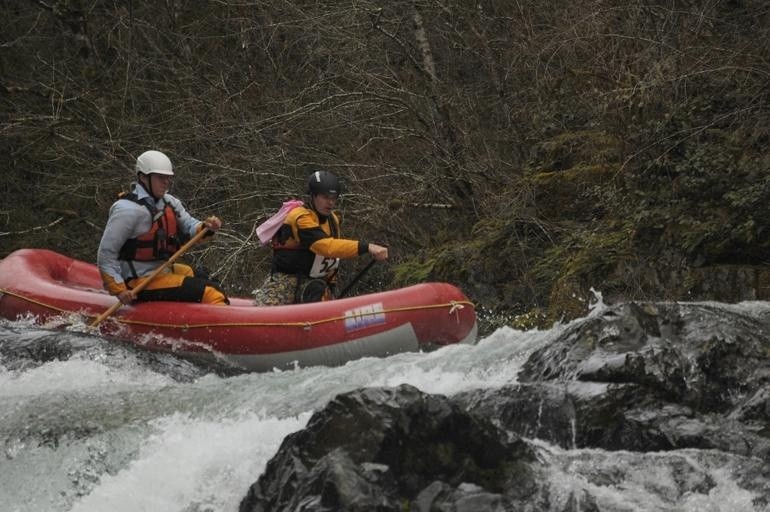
[96,150,231,305]
[270,170,389,304]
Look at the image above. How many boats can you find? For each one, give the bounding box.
[0,248,478,376]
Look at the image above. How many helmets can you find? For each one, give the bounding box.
[309,171,339,198]
[136,151,174,176]
